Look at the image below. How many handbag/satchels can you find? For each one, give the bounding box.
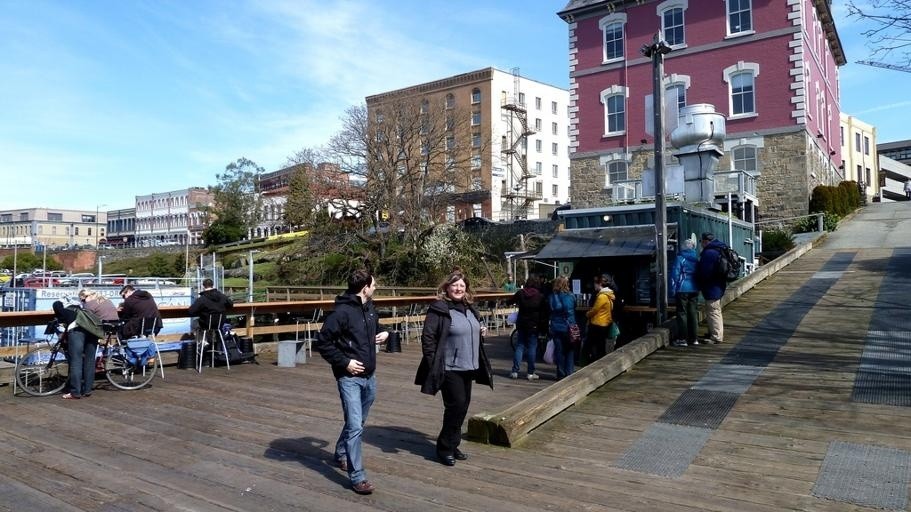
[76,309,106,339]
[124,338,156,368]
[543,341,555,364]
[570,322,582,342]
[508,312,518,322]
[609,322,621,338]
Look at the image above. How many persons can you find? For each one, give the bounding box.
[668,238,702,347]
[78,289,120,338]
[188,279,234,352]
[903,176,911,200]
[10,275,17,287]
[118,285,163,341]
[582,275,616,362]
[52,301,98,399]
[317,268,394,494]
[547,275,577,380]
[414,271,494,468]
[506,274,544,380]
[697,232,730,344]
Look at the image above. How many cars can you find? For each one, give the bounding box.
[456,217,500,227]
[158,238,181,246]
[368,222,403,234]
[0,268,177,288]
[55,244,114,251]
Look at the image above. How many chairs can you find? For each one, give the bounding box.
[195,312,231,375]
[12,318,59,396]
[293,304,324,359]
[123,314,167,382]
[392,296,519,346]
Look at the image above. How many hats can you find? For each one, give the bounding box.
[509,372,517,378]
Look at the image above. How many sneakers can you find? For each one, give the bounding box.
[671,338,719,347]
[528,374,539,380]
[336,457,348,471]
[352,480,375,493]
[440,449,468,465]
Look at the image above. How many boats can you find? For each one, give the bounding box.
[239,314,279,326]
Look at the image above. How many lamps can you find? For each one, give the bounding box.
[603,215,613,225]
[816,133,845,170]
[640,139,648,144]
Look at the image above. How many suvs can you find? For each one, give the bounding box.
[551,204,571,219]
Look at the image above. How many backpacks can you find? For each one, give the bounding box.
[711,246,740,281]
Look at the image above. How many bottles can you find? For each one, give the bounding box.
[583,293,593,307]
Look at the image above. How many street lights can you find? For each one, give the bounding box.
[98,255,107,283]
[640,29,674,323]
[248,249,261,303]
[96,203,107,250]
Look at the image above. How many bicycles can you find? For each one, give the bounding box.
[510,328,536,355]
[14,293,159,396]
